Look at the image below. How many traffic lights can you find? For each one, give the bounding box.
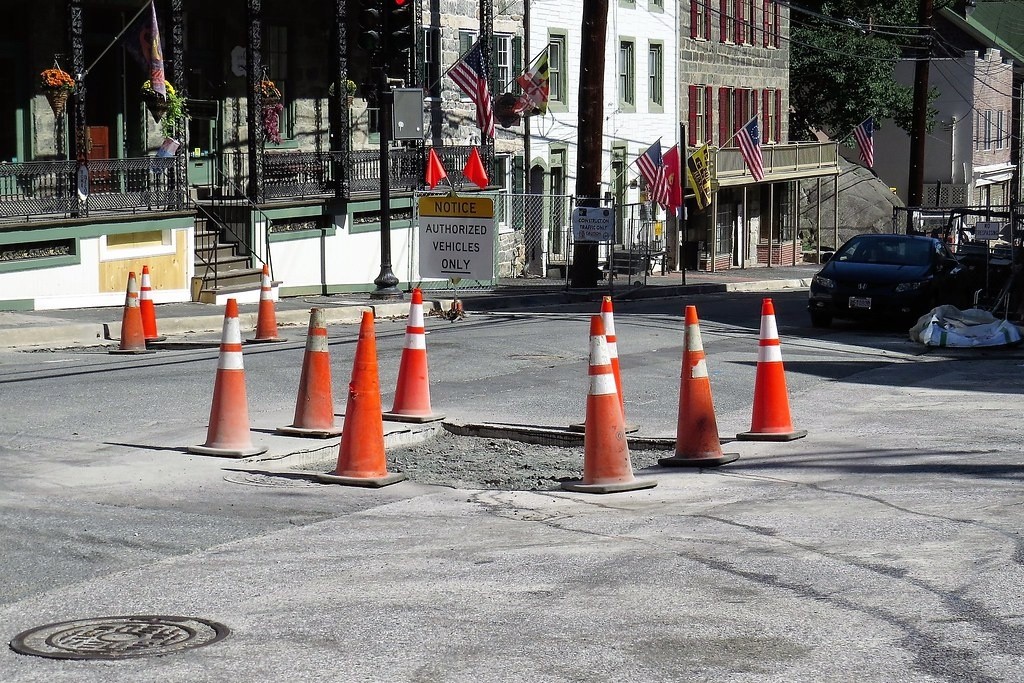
[356,0,385,57]
[387,0,417,54]
[391,89,427,140]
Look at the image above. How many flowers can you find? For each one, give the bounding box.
[139,79,194,140]
[37,69,76,94]
[256,80,285,146]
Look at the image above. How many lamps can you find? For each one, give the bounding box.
[631,179,647,191]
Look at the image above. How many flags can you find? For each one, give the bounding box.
[661,145,681,214]
[448,42,494,137]
[121,0,167,103]
[687,144,711,208]
[737,117,764,181]
[636,140,669,210]
[463,147,488,188]
[853,116,874,168]
[516,49,550,116]
[426,149,447,190]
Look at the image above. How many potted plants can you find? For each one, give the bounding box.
[329,80,357,106]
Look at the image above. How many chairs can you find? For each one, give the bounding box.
[864,243,886,257]
[917,250,929,259]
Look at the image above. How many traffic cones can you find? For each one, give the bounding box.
[567,296,644,435]
[559,314,660,498]
[240,263,289,341]
[272,309,345,437]
[380,288,448,423]
[313,310,412,488]
[136,266,172,345]
[183,298,274,461]
[656,303,745,467]
[105,270,155,356]
[734,299,824,442]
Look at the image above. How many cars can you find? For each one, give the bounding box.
[807,233,965,334]
